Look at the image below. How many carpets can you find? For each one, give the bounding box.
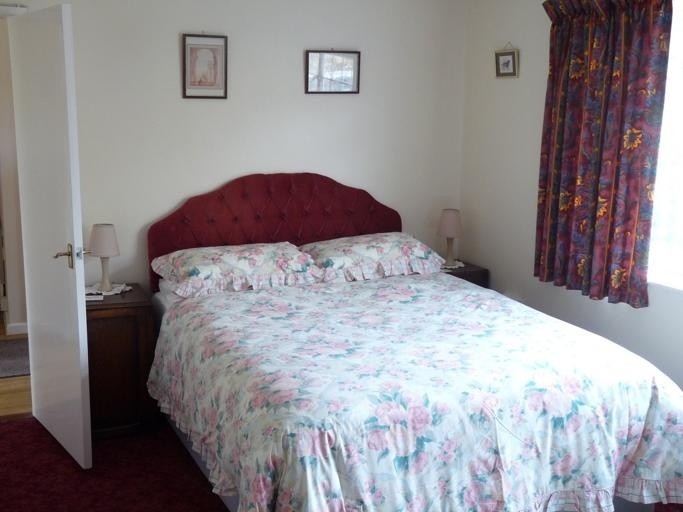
[0,412,232,512]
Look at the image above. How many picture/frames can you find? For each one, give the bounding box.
[494,49,518,77]
[304,50,361,94]
[182,32,227,100]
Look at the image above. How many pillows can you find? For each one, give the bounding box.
[150,232,446,303]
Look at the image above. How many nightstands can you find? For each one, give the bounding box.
[85,284,159,443]
[442,258,488,288]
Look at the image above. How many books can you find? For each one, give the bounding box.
[85,286,104,301]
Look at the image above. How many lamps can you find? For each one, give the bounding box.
[436,208,462,266]
[85,223,120,292]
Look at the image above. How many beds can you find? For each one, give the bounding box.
[145,172,670,512]
